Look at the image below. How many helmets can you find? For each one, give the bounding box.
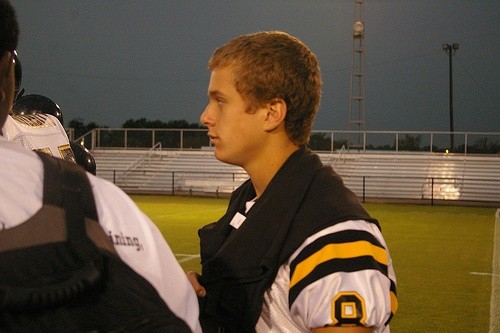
[69,141,96,177]
[12,94,63,126]
[13,51,22,106]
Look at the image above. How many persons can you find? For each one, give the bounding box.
[0,0,203,332]
[184,29,401,332]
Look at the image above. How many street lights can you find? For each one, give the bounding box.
[442,43,460,151]
